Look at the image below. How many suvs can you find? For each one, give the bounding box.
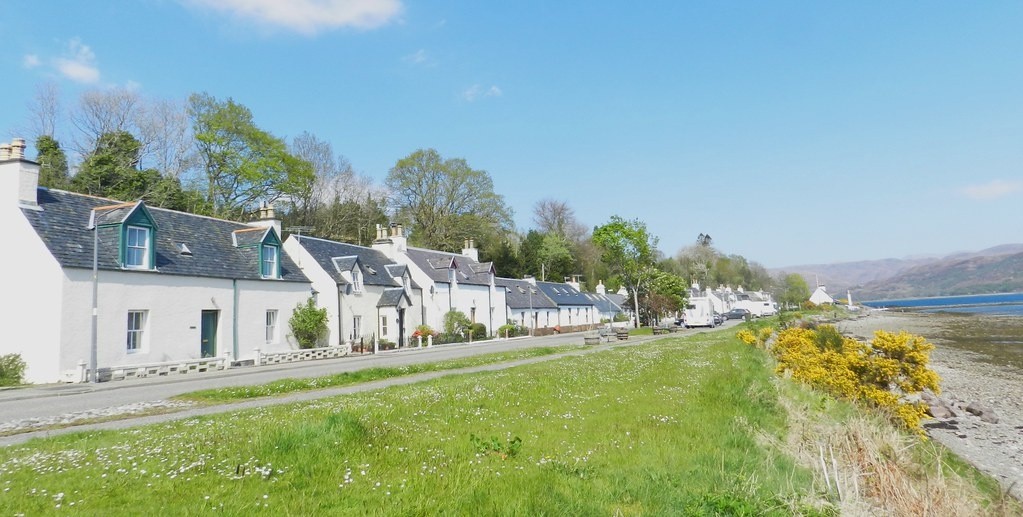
[721,307,751,321]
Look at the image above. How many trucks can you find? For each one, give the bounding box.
[682,296,715,329]
[735,299,774,319]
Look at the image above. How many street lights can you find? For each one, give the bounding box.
[608,288,613,329]
[90,204,126,385]
[527,284,534,336]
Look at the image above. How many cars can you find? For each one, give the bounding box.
[713,311,720,325]
[675,318,685,329]
